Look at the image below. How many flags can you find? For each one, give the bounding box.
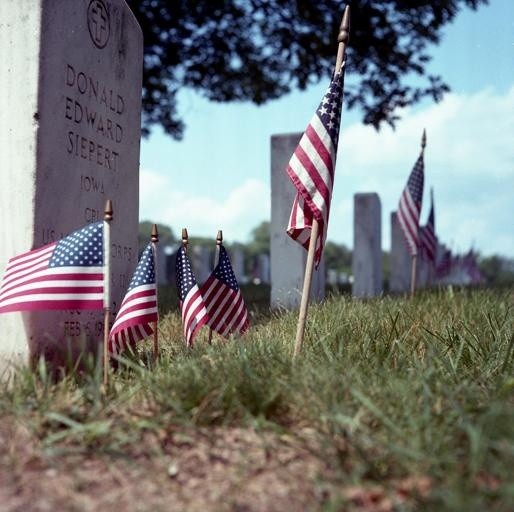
[394,154,425,257]
[287,44,345,271]
[176,245,210,347]
[432,245,491,288]
[106,239,158,357]
[0,220,105,314]
[200,244,251,342]
[420,201,437,260]
